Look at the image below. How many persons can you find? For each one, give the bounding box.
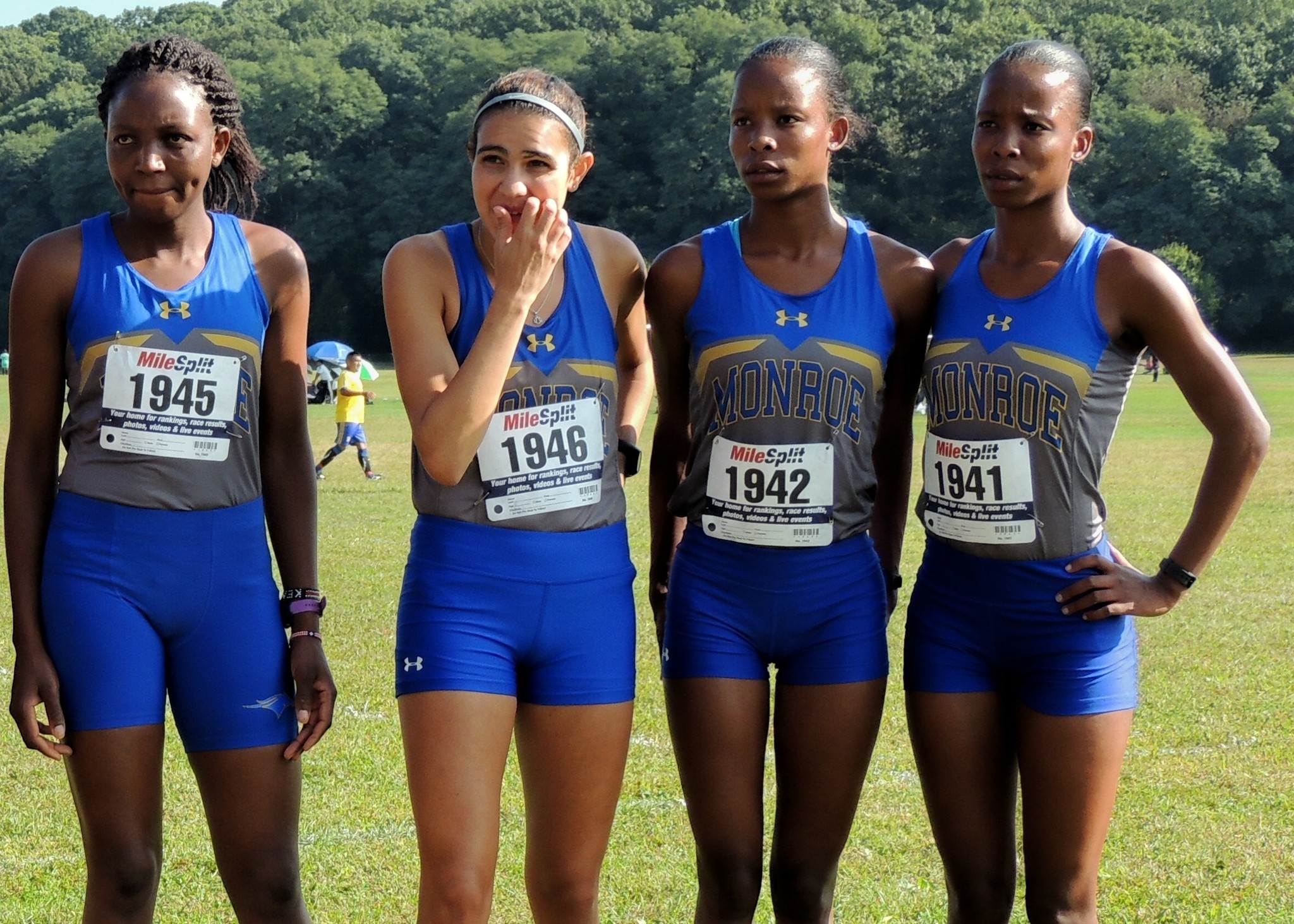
[4,34,338,924]
[380,68,649,924]
[902,43,1273,924]
[315,352,384,481]
[307,359,338,405]
[642,36,937,924]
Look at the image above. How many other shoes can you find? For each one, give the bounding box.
[316,473,326,480]
[366,473,384,480]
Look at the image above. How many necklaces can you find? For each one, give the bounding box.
[477,219,556,324]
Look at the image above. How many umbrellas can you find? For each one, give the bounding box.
[337,358,379,381]
[307,341,354,363]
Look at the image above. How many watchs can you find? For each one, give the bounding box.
[617,439,642,478]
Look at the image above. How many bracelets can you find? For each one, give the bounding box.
[1159,558,1197,588]
[281,587,321,600]
[288,631,322,645]
[280,596,327,628]
[881,569,902,589]
